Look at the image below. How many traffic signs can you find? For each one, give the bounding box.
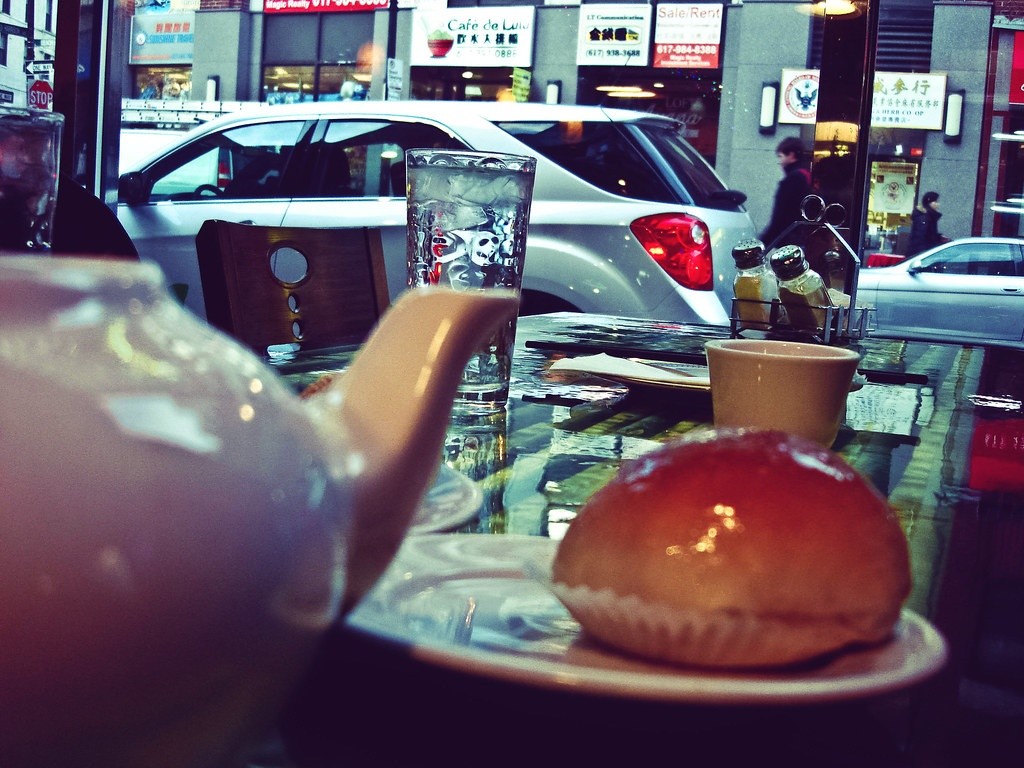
[0,89,14,104]
[26,63,54,75]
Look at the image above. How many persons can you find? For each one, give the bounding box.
[904,190,943,258]
[755,134,833,270]
[0,115,140,263]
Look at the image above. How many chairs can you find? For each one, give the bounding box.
[196,219,391,355]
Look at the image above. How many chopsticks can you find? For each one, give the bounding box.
[525,341,928,385]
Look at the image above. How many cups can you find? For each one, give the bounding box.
[702,336,861,461]
[404,146,537,403]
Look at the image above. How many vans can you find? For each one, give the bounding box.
[79,131,238,197]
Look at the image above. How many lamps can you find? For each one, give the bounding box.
[942,88,966,145]
[758,81,780,135]
[545,79,562,105]
[206,75,220,101]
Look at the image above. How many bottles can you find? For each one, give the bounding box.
[771,245,836,329]
[733,240,778,329]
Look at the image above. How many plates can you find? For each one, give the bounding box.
[406,461,484,536]
[335,528,951,707]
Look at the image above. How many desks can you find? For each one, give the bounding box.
[258,311,987,767]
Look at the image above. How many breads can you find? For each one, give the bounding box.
[546,424,911,667]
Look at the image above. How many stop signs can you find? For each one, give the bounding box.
[27,79,54,113]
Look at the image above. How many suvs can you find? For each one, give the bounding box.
[110,102,770,370]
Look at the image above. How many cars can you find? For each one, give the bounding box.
[851,234,1024,352]
